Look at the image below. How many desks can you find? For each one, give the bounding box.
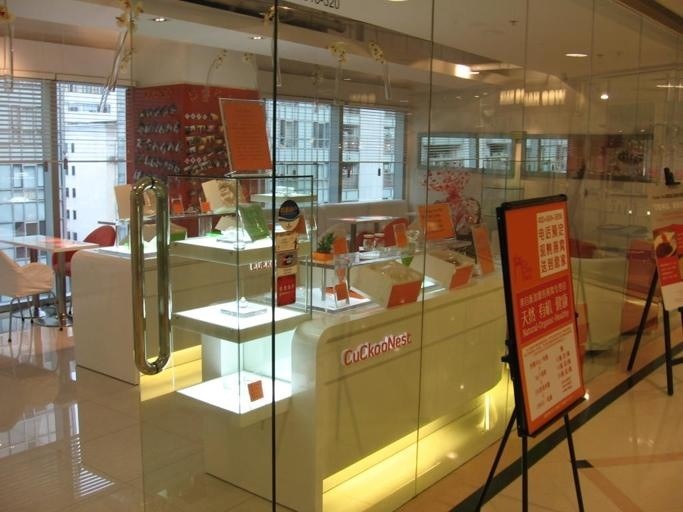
[0,235,99,325]
[594,224,650,256]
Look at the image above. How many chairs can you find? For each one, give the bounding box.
[0,249,64,341]
[52,226,116,314]
[569,257,625,360]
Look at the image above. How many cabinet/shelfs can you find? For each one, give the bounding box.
[71,176,580,512]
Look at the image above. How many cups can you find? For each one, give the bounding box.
[361,234,384,251]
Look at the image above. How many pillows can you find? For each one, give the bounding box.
[571,235,597,259]
